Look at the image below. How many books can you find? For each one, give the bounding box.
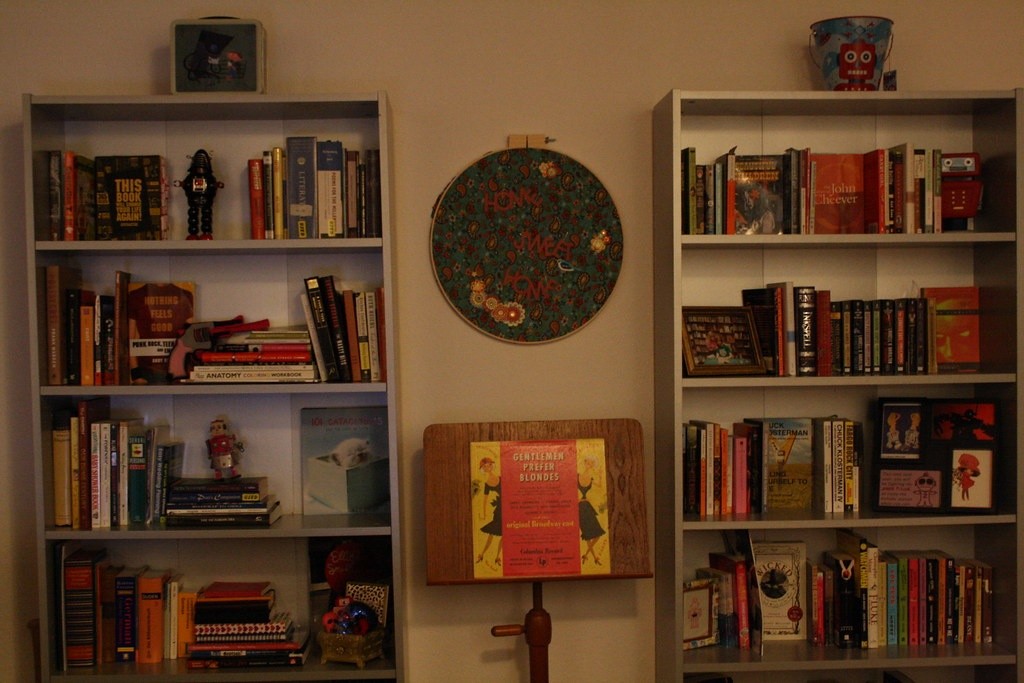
[42,136,387,669]
[679,147,997,652]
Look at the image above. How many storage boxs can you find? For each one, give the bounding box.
[95,154,168,241]
[170,16,268,94]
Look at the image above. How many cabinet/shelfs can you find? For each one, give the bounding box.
[23,92,404,683]
[652,88,1024,683]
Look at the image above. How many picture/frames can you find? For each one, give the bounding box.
[682,306,768,377]
[870,465,953,515]
[872,396,929,460]
